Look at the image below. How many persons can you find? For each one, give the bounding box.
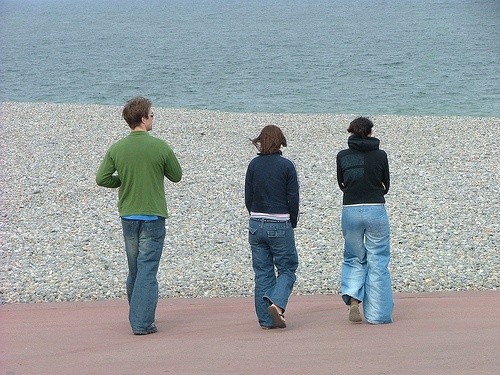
[337,118,394,324]
[245,125,299,329]
[95,95,182,335]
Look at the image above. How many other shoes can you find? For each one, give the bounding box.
[133,324,158,334]
[347,304,362,322]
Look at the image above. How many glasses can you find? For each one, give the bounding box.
[145,111,154,117]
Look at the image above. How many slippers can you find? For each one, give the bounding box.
[269,304,287,328]
[261,325,274,329]
